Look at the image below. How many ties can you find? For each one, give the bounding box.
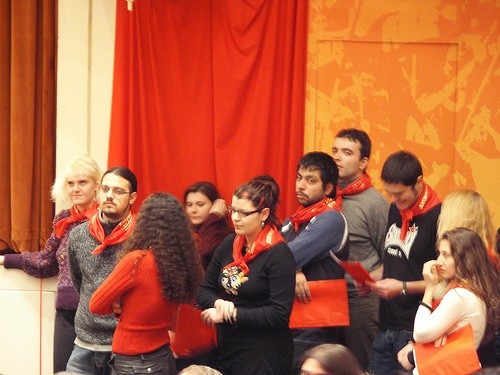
[53,200,98,238]
[223,223,286,276]
[400,181,441,240]
[289,196,342,231]
[335,172,372,204]
[89,212,136,255]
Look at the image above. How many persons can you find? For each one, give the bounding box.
[333,129,390,375]
[0,154,112,375]
[90,192,201,375]
[196,182,296,375]
[397,188,500,372]
[413,227,500,375]
[181,364,223,375]
[66,166,137,375]
[251,175,283,232]
[183,182,234,276]
[354,150,442,375]
[280,151,350,375]
[299,343,367,375]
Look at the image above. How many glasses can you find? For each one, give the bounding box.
[227,205,261,217]
[97,186,129,195]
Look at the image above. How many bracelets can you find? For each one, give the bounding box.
[401,281,409,298]
